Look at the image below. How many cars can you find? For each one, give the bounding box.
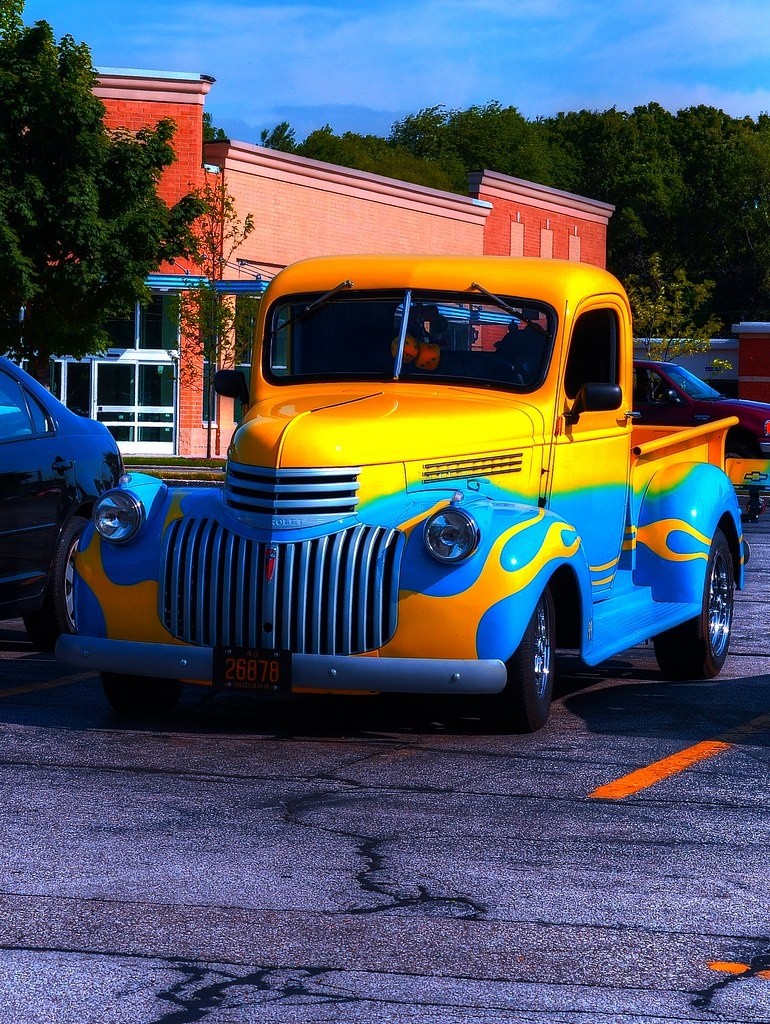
[50,254,749,739]
[0,352,135,657]
[632,359,770,463]
